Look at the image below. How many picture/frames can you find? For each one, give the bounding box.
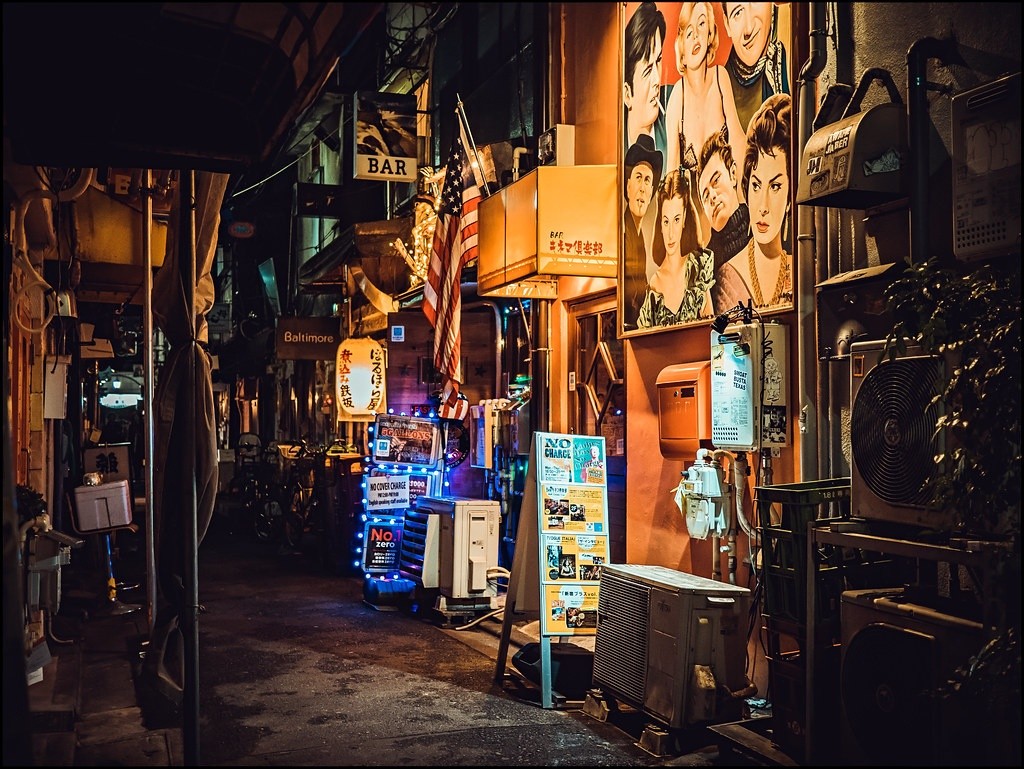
[615,2,794,339]
[420,357,467,386]
[372,413,440,469]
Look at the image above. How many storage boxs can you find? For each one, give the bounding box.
[763,615,836,764]
[416,495,501,599]
[754,479,853,614]
[77,480,131,532]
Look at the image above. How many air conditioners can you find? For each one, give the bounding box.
[837,590,1002,769]
[845,338,1010,536]
[591,562,751,727]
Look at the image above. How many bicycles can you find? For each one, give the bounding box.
[229,435,348,565]
